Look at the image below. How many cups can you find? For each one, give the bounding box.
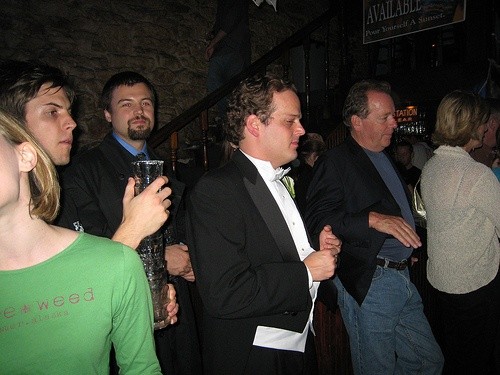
[132,160,164,195]
[135,233,170,330]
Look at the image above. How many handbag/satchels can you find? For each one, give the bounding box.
[413,175,428,229]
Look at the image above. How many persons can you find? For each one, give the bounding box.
[306,81,445,375]
[1,107,164,374]
[205,0,248,128]
[0,62,182,331]
[57,71,200,375]
[293,99,500,232]
[187,71,343,375]
[419,89,500,375]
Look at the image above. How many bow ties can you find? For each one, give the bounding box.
[271,166,291,182]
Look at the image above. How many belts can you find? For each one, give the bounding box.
[377,256,409,270]
